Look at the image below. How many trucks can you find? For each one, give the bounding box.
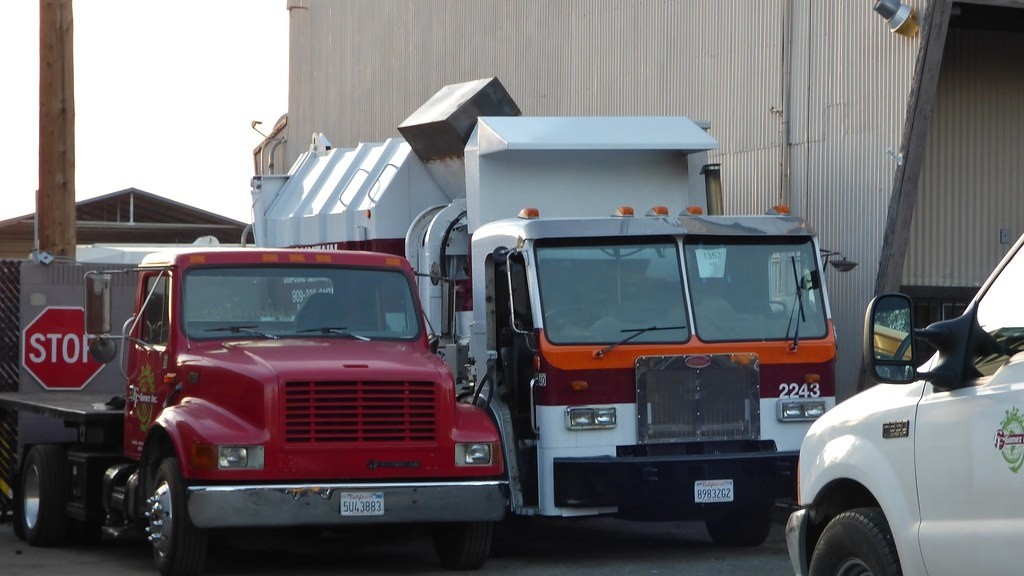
[249,77,859,550]
[0,234,511,576]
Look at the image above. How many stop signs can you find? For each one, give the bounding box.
[22,303,106,392]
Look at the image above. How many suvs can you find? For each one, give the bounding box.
[782,227,1024,576]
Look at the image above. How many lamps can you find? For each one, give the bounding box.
[872,0,912,33]
